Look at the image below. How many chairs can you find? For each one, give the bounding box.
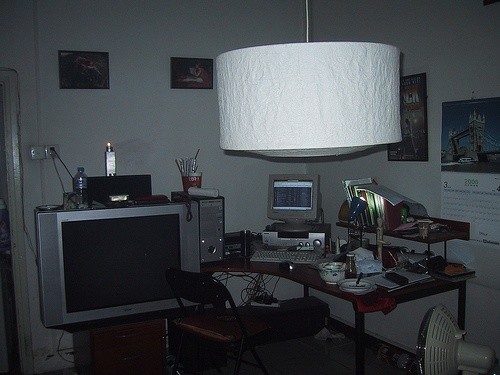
[165,269,272,375]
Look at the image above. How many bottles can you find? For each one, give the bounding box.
[74,167,88,209]
[345,253,357,279]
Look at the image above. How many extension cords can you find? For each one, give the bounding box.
[249,300,281,307]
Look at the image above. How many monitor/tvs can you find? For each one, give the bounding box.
[35,201,202,332]
[266,174,319,232]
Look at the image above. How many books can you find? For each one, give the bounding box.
[343,178,409,231]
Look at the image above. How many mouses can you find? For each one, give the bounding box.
[279,261,296,270]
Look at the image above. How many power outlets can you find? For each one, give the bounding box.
[45,145,58,159]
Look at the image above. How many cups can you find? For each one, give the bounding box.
[182,171,203,191]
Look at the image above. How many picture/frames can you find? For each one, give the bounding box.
[388,72,429,161]
[170,57,213,90]
[59,50,110,90]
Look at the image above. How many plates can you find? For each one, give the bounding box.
[336,279,378,295]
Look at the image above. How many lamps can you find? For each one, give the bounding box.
[215,0,403,157]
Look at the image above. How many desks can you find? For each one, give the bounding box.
[200,255,476,375]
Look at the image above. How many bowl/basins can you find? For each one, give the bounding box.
[318,262,346,284]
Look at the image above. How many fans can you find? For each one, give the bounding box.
[416,304,496,375]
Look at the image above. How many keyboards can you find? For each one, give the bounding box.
[250,249,321,264]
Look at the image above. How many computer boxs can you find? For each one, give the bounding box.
[171,190,225,263]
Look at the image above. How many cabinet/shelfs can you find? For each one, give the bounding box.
[72,317,167,375]
